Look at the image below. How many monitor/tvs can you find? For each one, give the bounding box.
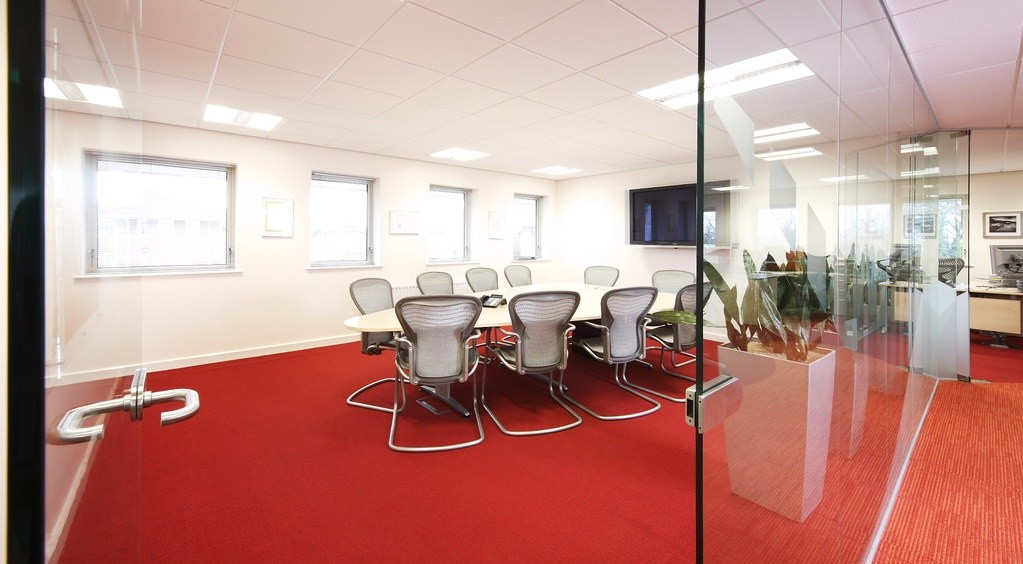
[629,183,696,245]
[989,245,1023,281]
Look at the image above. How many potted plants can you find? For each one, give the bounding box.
[831,242,891,462]
[650,248,836,523]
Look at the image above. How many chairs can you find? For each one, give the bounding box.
[347,265,714,452]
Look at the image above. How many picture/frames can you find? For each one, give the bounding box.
[904,214,937,240]
[983,211,1023,239]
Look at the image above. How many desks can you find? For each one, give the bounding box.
[874,278,923,335]
[969,284,1022,348]
[344,282,677,416]
